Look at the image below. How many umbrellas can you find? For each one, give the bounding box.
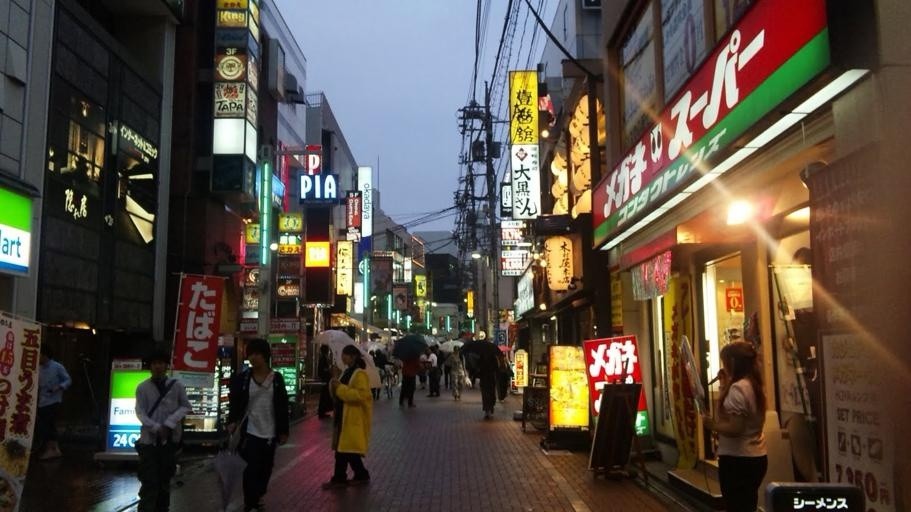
[211,430,250,511]
[310,329,383,391]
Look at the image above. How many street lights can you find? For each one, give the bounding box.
[471,249,500,347]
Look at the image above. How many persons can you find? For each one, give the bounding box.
[134,340,194,511]
[360,329,504,420]
[226,336,291,511]
[28,342,74,461]
[319,343,376,489]
[702,340,769,511]
[316,343,335,421]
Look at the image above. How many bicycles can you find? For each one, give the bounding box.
[383,366,398,401]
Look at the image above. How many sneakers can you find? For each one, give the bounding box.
[485,408,494,417]
[398,402,416,407]
[455,395,461,400]
[427,393,440,397]
[323,476,347,488]
[347,471,370,486]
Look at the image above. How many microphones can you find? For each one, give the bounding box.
[705,369,725,385]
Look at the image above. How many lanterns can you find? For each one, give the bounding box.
[543,235,575,292]
